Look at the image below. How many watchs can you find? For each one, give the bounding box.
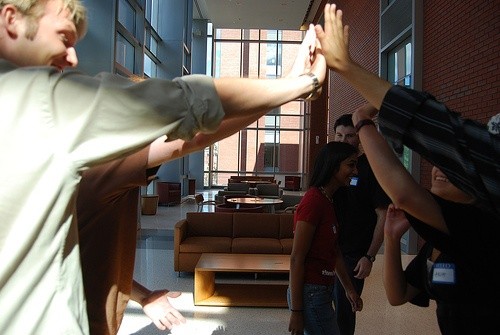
[365,254,376,262]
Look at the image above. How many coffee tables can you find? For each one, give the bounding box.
[194,253,291,308]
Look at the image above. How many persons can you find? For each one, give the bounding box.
[76,23,326,335]
[287,3,500,335]
[0,0,327,335]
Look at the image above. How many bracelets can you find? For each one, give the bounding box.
[298,73,320,102]
[290,309,305,312]
[355,119,377,131]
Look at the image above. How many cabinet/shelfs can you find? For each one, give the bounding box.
[157,182,181,207]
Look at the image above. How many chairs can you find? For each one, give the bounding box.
[216,204,264,213]
[223,195,257,204]
[194,194,216,212]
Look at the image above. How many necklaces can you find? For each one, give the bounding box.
[320,184,334,204]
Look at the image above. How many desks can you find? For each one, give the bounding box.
[227,198,283,212]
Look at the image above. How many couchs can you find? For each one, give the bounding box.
[174,213,293,279]
[224,183,254,195]
[228,176,276,188]
[254,183,283,199]
